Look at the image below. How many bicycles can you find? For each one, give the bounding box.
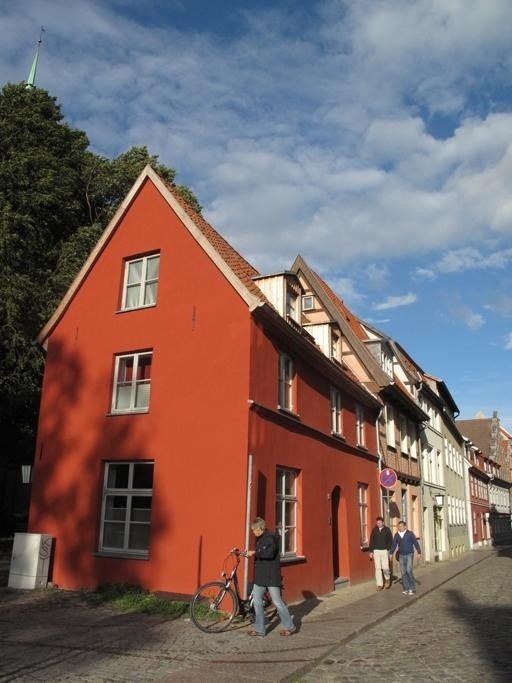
[190,545,285,636]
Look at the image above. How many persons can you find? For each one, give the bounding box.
[246,516,299,638]
[389,520,422,596]
[368,516,393,591]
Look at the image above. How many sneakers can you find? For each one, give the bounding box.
[408,589,416,595]
[402,590,409,595]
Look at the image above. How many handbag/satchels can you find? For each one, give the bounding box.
[396,550,399,560]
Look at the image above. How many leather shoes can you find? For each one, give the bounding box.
[384,579,391,589]
[248,630,265,638]
[280,627,295,635]
[376,585,382,591]
